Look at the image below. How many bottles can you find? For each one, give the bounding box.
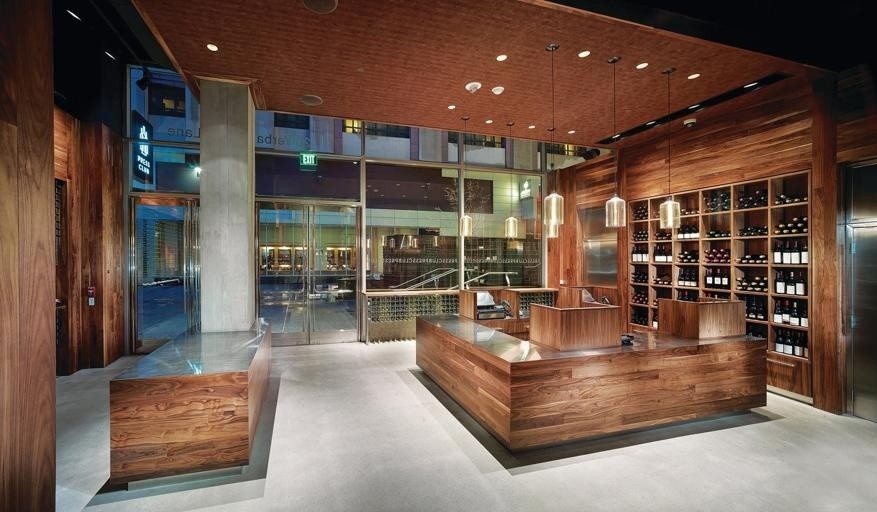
[629,189,809,363]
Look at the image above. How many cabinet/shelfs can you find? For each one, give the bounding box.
[625,168,814,406]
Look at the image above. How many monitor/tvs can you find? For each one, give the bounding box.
[476,290,495,307]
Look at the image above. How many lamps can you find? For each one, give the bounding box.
[607,53,628,232]
[544,42,565,238]
[366,205,439,250]
[460,114,473,238]
[505,120,519,237]
[658,66,682,232]
